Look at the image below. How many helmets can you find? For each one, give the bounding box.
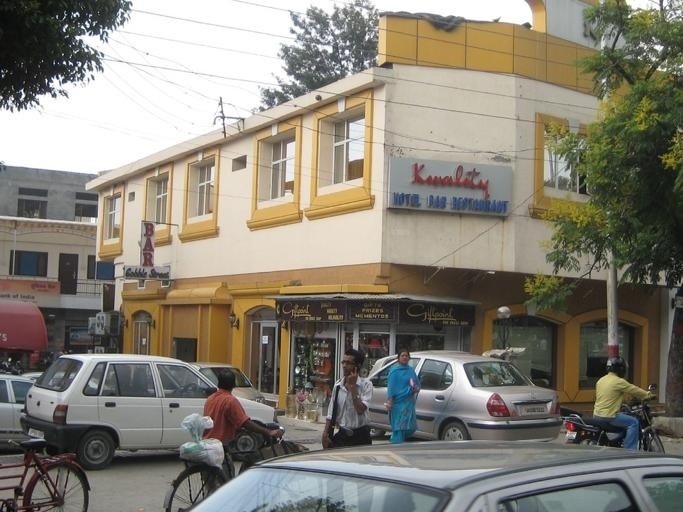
[607,356,626,377]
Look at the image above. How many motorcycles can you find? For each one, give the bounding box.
[560,385,664,453]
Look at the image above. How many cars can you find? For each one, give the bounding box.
[369,353,562,441]
[24,372,105,394]
[0,376,35,442]
[185,441,681,510]
[27,352,275,469]
[189,364,264,404]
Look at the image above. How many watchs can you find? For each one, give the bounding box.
[353,394,360,401]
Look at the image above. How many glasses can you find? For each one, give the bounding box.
[341,360,355,365]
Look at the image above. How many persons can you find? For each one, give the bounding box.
[197,369,285,496]
[592,355,657,450]
[383,347,422,445]
[319,347,375,512]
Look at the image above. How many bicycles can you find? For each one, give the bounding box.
[0,439,90,512]
[159,414,305,512]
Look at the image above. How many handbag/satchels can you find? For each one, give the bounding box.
[239,441,309,475]
[328,429,345,447]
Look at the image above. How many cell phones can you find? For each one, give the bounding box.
[353,365,360,374]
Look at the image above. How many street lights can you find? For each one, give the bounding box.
[496,307,514,349]
